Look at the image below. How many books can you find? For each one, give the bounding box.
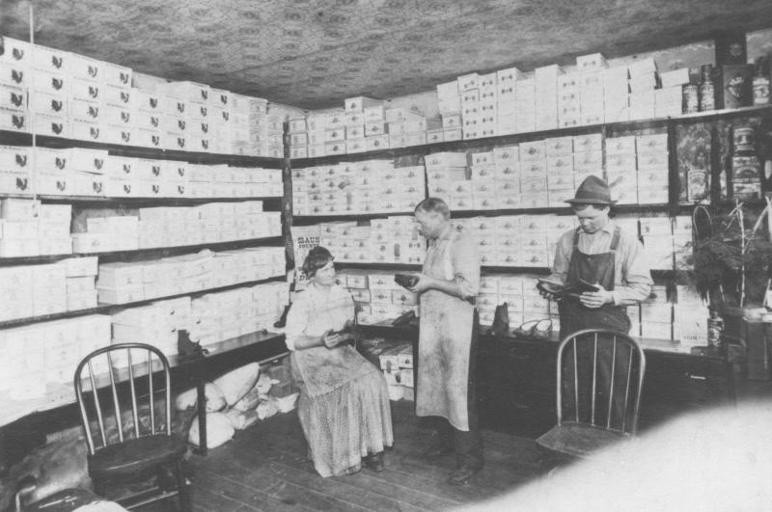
[537,276,599,303]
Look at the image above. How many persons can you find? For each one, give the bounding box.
[284,246,395,478]
[402,197,484,486]
[534,174,654,431]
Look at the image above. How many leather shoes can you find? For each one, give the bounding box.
[450,466,479,486]
[367,453,384,473]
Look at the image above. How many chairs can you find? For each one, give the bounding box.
[75,342,188,511]
[535,328,647,477]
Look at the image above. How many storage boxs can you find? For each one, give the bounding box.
[379,345,414,401]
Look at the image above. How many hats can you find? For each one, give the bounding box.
[564,175,618,205]
